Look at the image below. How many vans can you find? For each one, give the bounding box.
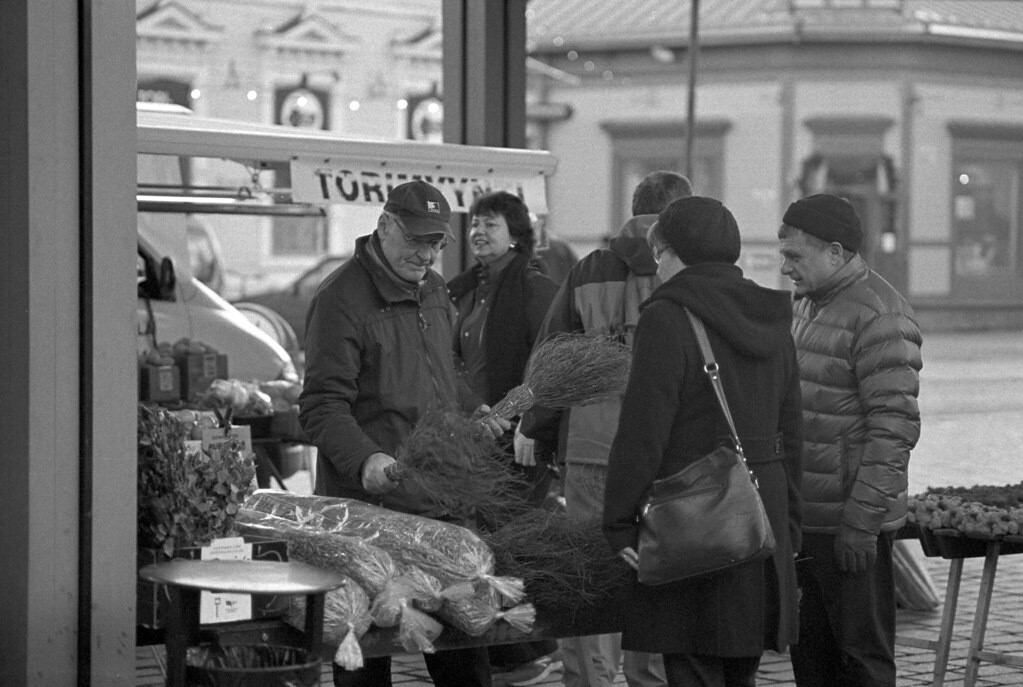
[137,230,304,413]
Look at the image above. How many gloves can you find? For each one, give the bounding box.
[833,519,878,576]
[534,434,562,479]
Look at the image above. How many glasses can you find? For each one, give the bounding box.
[653,245,672,264]
[393,219,448,250]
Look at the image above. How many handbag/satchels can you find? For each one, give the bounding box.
[637,305,777,591]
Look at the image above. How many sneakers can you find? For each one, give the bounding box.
[502,649,565,687]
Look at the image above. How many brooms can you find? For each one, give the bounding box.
[351,328,634,621]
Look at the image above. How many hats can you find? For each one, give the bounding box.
[384,182,457,243]
[659,195,741,267]
[782,193,863,252]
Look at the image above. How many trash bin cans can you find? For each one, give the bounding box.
[137,558,350,687]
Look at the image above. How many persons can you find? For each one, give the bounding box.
[443,189,566,535]
[776,195,924,686]
[519,171,694,686]
[600,195,806,686]
[296,181,514,520]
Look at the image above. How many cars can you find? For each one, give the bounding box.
[236,253,353,350]
